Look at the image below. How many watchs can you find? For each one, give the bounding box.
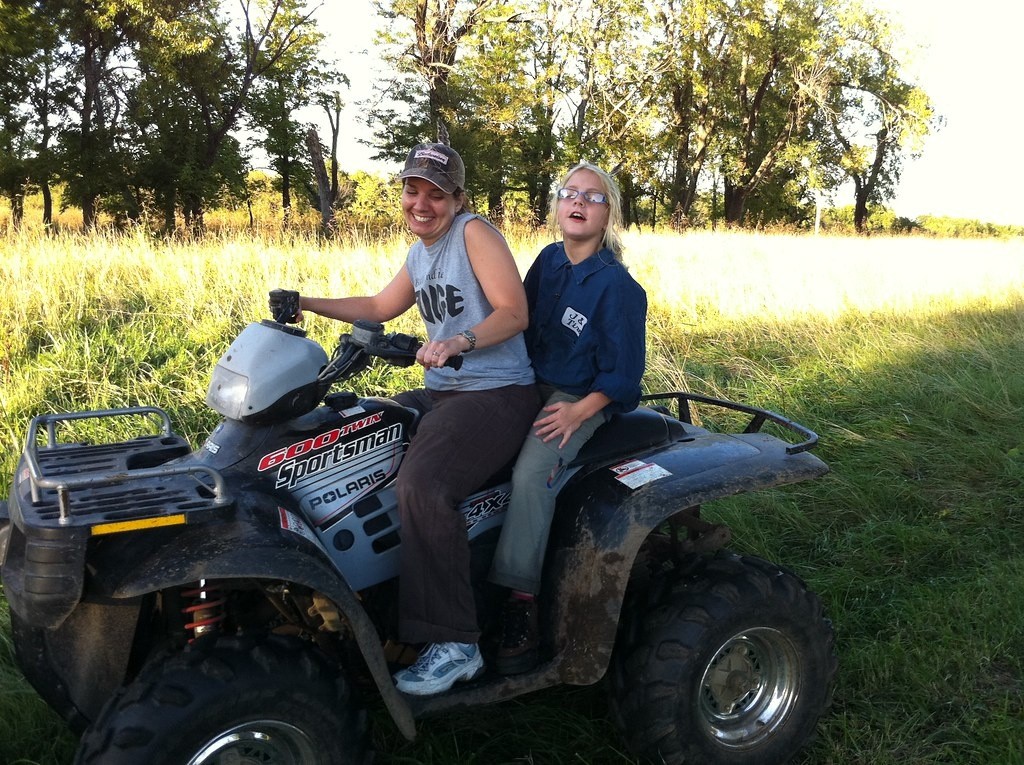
[457,330,477,353]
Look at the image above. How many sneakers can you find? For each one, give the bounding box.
[391,641,485,700]
[494,596,553,675]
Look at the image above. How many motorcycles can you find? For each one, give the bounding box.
[0,289,842,765]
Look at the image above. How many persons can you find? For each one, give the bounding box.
[268,143,541,695]
[487,163,647,675]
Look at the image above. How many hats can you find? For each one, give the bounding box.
[398,143,465,194]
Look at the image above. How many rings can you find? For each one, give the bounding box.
[433,352,440,356]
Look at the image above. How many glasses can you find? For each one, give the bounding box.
[556,188,611,208]
